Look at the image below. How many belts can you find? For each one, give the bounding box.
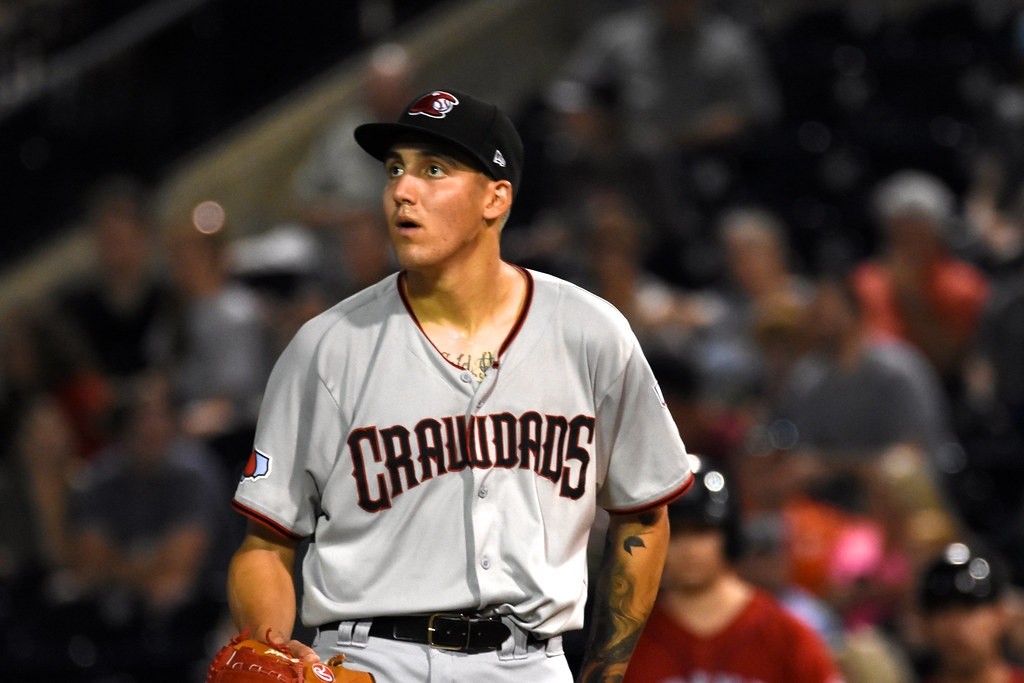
[318,609,548,655]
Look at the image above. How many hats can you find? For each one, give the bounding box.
[354,88,523,201]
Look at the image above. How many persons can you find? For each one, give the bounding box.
[1,0,1024,683]
[207,88,695,683]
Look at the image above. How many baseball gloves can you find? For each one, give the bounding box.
[207,626,378,682]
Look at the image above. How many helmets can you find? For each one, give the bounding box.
[669,455,746,561]
[916,546,1004,612]
